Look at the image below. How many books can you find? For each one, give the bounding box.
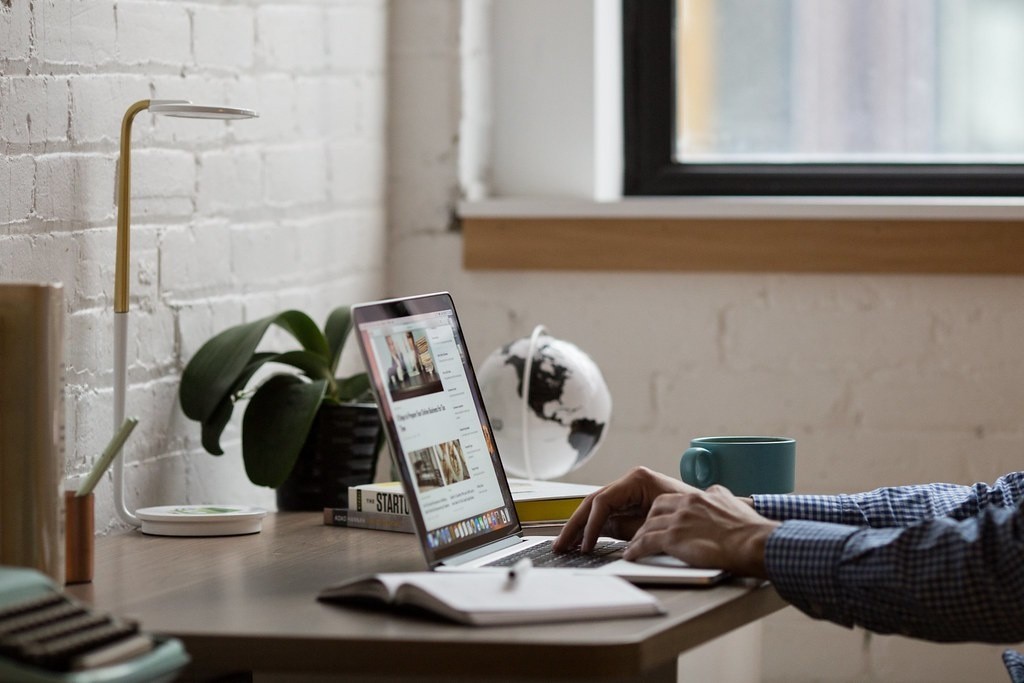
[314,573,675,631]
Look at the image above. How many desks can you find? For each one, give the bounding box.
[59,480,788,683]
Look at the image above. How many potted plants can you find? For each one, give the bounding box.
[177,304,386,515]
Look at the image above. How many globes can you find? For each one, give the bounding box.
[477,323,613,483]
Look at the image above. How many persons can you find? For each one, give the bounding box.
[551,465,1024,683]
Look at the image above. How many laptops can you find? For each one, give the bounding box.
[351,292,736,585]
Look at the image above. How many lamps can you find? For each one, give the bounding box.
[111,99,264,537]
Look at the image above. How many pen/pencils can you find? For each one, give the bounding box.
[506,557,532,578]
[74,416,139,496]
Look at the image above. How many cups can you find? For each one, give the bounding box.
[679,436,796,498]
[64,490,95,587]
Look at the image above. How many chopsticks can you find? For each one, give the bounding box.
[76,416,138,497]
